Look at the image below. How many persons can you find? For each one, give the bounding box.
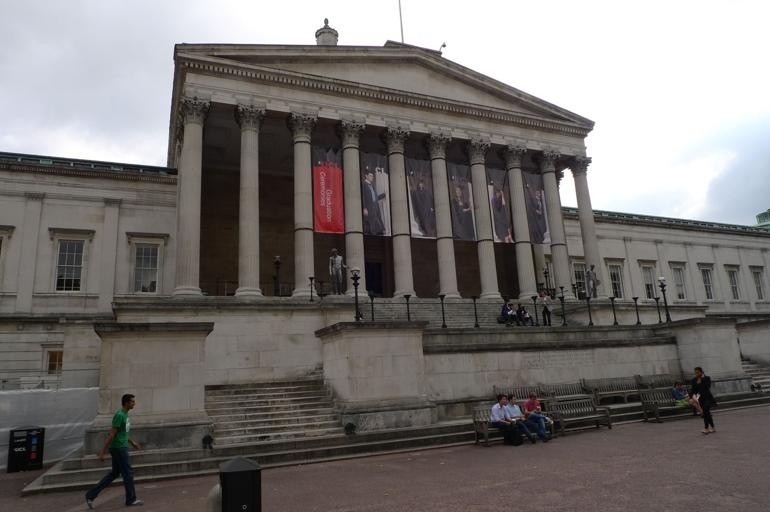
[501,301,534,327]
[527,189,547,243]
[492,190,514,243]
[85,394,145,510]
[584,264,598,299]
[360,171,387,236]
[491,392,554,446]
[671,381,713,419]
[687,367,716,433]
[328,248,349,296]
[411,181,436,237]
[453,186,471,239]
[541,291,553,326]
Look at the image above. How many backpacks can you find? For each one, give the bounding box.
[508,422,523,445]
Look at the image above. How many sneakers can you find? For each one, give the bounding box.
[132,499,144,505]
[532,434,552,443]
[85,494,93,508]
[702,429,716,433]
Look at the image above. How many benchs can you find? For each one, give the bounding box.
[470,372,698,447]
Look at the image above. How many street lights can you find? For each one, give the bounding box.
[658,277,672,323]
[542,265,549,296]
[272,256,281,296]
[350,266,360,321]
